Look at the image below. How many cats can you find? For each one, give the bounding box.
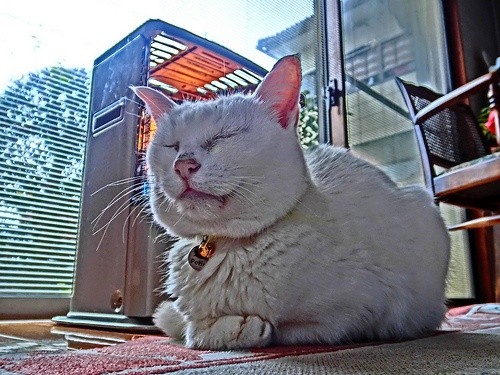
[126,51,451,350]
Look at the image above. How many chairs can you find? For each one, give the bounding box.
[395,60,500,321]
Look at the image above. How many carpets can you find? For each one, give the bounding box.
[0,301,500,375]
